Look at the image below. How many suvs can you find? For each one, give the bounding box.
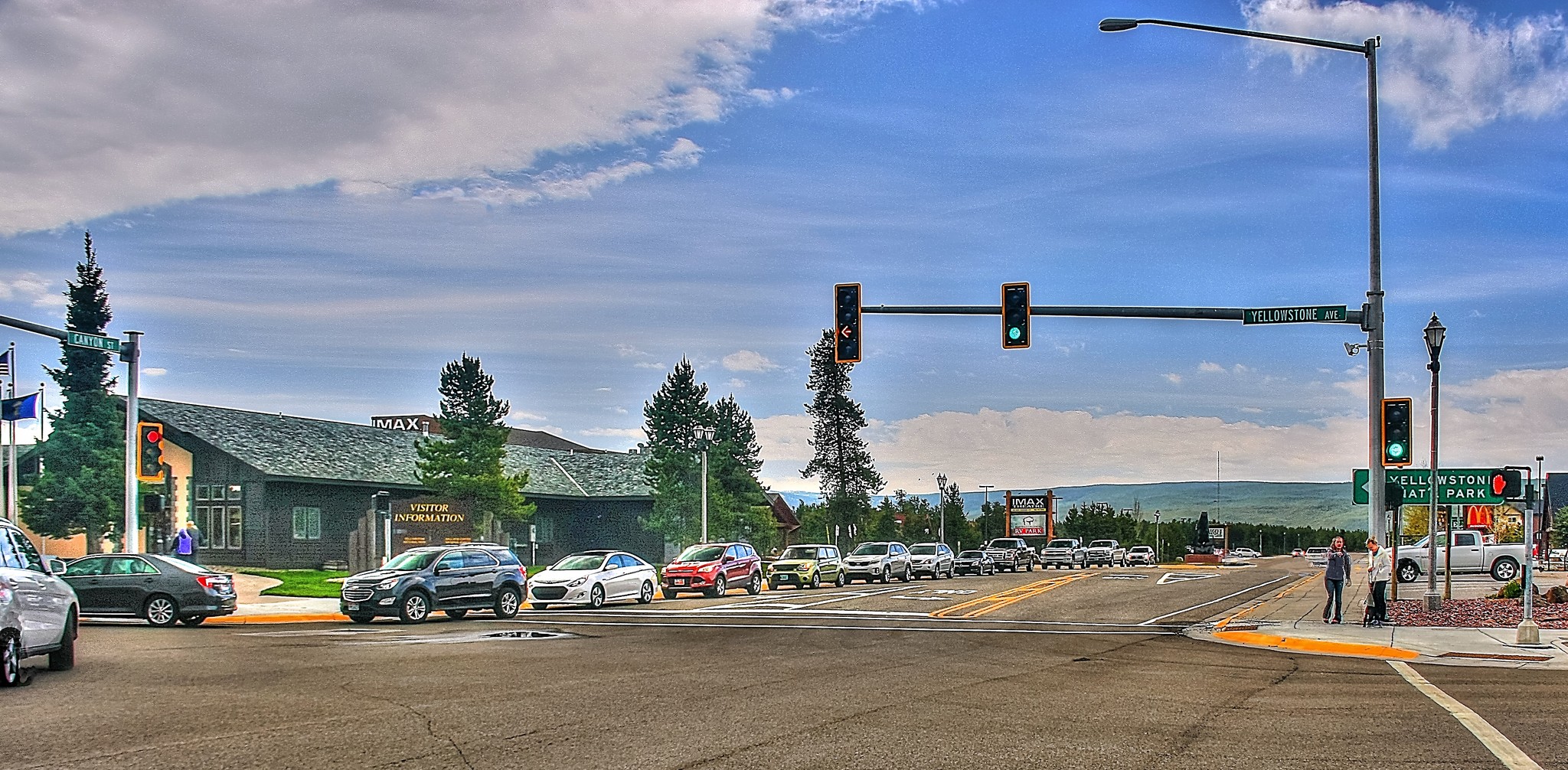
[906,541,957,579]
[336,542,528,624]
[1305,546,1331,568]
[0,515,82,685]
[659,540,763,596]
[766,542,845,590]
[1214,547,1262,559]
[842,540,914,583]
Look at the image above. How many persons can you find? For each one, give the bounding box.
[1323,537,1392,628]
[169,521,207,565]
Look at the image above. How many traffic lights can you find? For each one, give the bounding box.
[1001,283,1030,348]
[1491,471,1520,495]
[835,285,861,363]
[1382,399,1411,464]
[139,422,165,480]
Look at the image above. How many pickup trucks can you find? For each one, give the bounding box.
[1040,539,1089,570]
[978,537,1037,573]
[1084,539,1126,568]
[1384,530,1540,584]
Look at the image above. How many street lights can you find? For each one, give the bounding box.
[694,425,715,543]
[1422,311,1451,616]
[1097,16,1391,612]
[937,472,948,543]
[1153,510,1161,562]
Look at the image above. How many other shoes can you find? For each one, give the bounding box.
[1323,618,1386,626]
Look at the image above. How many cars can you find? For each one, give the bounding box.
[523,547,659,608]
[1124,545,1156,566]
[953,550,996,576]
[56,553,240,628]
[1291,548,1304,557]
